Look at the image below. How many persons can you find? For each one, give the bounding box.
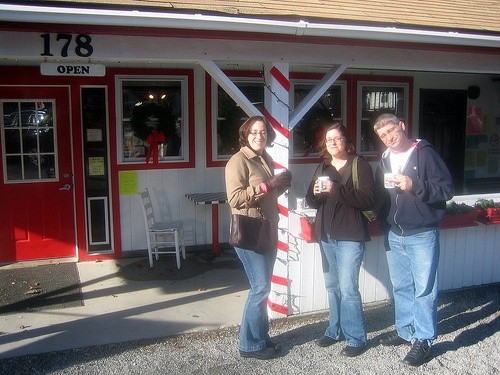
[305,122,375,356]
[225,115,292,361]
[372,113,455,366]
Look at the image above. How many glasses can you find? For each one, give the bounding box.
[325,139,344,143]
[249,132,268,137]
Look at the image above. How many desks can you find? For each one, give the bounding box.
[184,192,228,256]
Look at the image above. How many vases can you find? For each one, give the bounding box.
[367,220,385,236]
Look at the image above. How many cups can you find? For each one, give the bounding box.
[317,176,330,191]
[296,198,309,211]
[383,173,400,189]
[274,168,286,176]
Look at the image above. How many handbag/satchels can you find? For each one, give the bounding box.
[230,193,271,251]
[352,156,381,221]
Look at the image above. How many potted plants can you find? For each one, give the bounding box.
[439,199,482,230]
[299,216,318,244]
[472,197,500,226]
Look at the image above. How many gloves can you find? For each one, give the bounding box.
[265,170,293,190]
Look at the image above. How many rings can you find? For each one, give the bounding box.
[323,186,326,189]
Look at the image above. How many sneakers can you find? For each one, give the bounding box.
[341,344,368,356]
[379,336,408,345]
[403,339,430,366]
[315,335,335,346]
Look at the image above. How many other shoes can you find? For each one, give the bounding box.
[265,340,282,352]
[240,346,275,359]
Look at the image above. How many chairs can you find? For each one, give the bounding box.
[138,187,186,271]
